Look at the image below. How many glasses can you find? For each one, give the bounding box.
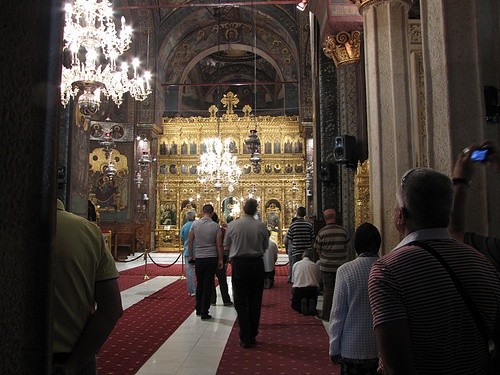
[401,168,416,208]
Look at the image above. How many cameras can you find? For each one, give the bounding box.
[470,147,493,162]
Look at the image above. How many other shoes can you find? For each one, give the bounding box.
[210,302,216,306]
[196,311,210,315]
[239,341,256,348]
[187,292,196,297]
[201,315,211,319]
[301,297,307,316]
[309,297,316,315]
[263,279,271,289]
[224,302,233,306]
[315,315,329,323]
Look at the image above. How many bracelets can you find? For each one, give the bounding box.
[451,177,468,185]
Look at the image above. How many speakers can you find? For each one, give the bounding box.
[56,164,66,184]
[333,135,357,163]
[319,161,336,181]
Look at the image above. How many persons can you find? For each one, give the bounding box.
[329,222,382,375]
[223,199,268,348]
[368,167,500,375]
[284,206,324,295]
[188,204,223,319]
[263,230,278,289]
[313,209,350,322]
[449,137,500,273]
[291,250,321,316]
[162,204,172,224]
[180,210,240,306]
[51,175,123,375]
